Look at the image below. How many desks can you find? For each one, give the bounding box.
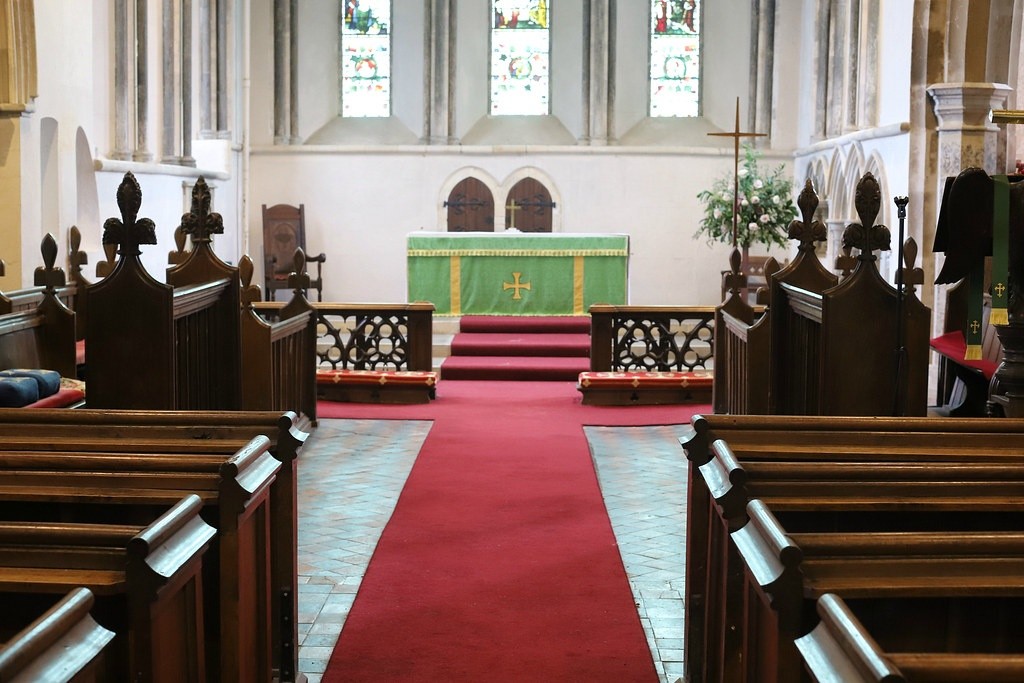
[405,231,629,317]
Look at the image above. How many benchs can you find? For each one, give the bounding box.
[0,225,90,409]
[317,370,437,403]
[0,407,310,683]
[575,371,713,404]
[679,413,1024,683]
[437,165,561,232]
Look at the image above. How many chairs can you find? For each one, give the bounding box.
[721,256,789,304]
[262,204,327,302]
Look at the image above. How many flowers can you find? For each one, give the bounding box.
[693,143,798,251]
